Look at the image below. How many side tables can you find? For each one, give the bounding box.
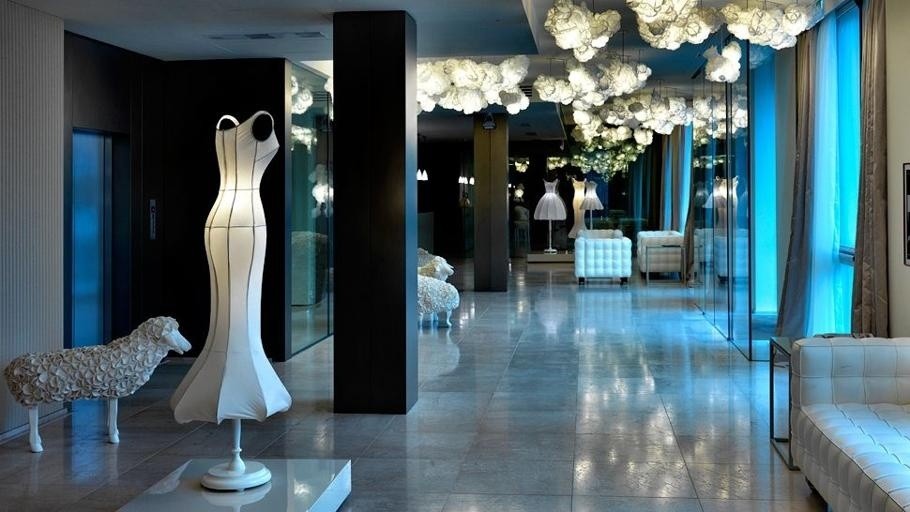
[768,334,852,472]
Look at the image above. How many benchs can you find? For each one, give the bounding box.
[789,338,910,511]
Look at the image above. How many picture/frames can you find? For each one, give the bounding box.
[903,163,910,266]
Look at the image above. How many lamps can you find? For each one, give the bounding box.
[631,0,720,55]
[418,54,530,120]
[542,0,622,62]
[699,39,743,85]
[533,50,654,111]
[720,1,821,45]
[566,94,685,149]
[685,92,748,144]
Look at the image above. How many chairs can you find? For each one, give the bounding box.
[574,229,685,286]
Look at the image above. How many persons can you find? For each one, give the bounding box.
[534,178,567,221]
[580,180,603,212]
[568,176,588,238]
[168,110,293,424]
